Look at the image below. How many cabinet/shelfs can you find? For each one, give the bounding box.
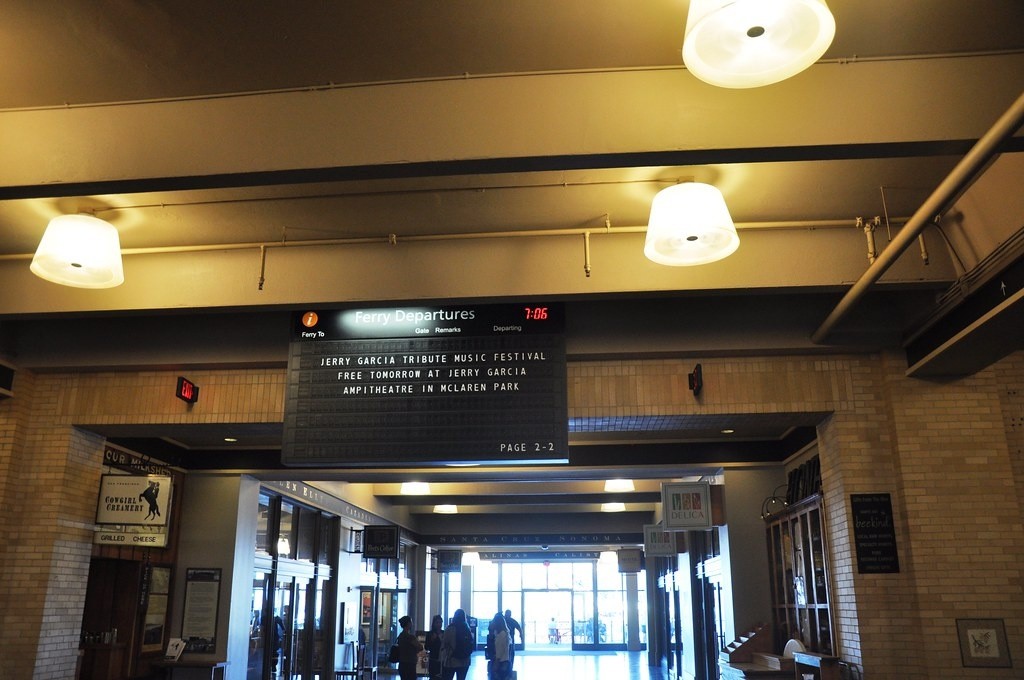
[793,652,840,680]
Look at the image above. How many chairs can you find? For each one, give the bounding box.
[335,640,378,680]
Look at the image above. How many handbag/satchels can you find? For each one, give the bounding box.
[388,638,399,663]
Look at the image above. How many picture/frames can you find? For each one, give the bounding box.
[361,590,384,627]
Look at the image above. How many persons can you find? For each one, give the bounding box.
[442,609,473,680]
[251,610,260,656]
[271,605,293,672]
[398,616,423,680]
[424,615,445,680]
[488,610,523,680]
[548,618,559,644]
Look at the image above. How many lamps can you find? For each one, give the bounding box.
[643,176,741,267]
[676,0,837,90]
[27,205,125,290]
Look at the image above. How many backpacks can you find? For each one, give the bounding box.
[448,623,473,659]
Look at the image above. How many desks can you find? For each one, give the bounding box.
[156,658,231,680]
